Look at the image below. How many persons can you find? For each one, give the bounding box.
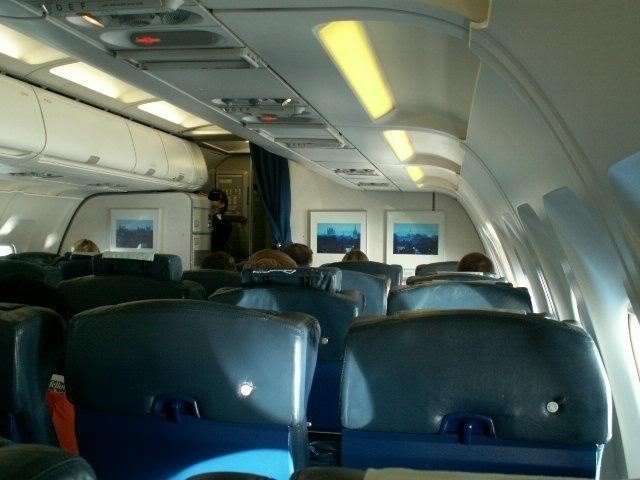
[242,248,299,269]
[200,187,234,251]
[280,243,314,267]
[457,251,495,272]
[342,248,370,262]
[71,237,101,254]
[198,252,237,270]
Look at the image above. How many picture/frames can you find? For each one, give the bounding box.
[109,209,159,251]
[386,210,447,270]
[312,212,367,265]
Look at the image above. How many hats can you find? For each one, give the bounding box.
[242,249,297,270]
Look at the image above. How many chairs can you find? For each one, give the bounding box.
[0,250,612,479]
